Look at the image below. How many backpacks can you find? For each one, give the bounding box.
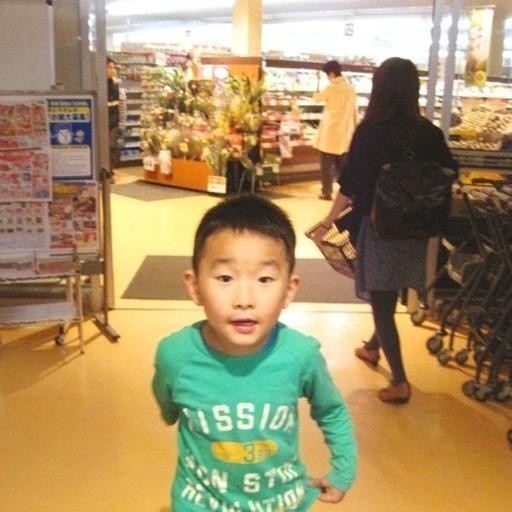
[371,118,448,240]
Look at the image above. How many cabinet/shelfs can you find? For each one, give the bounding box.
[101,53,512,194]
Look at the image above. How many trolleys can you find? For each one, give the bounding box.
[408,176,512,404]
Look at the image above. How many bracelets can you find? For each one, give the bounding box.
[321,223,334,230]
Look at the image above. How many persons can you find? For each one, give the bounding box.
[312,59,358,202]
[106,55,120,185]
[310,56,461,402]
[151,193,359,512]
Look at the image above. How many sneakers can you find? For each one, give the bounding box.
[356,345,379,365]
[379,381,411,402]
[319,193,332,200]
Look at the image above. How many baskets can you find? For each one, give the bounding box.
[305,200,358,279]
